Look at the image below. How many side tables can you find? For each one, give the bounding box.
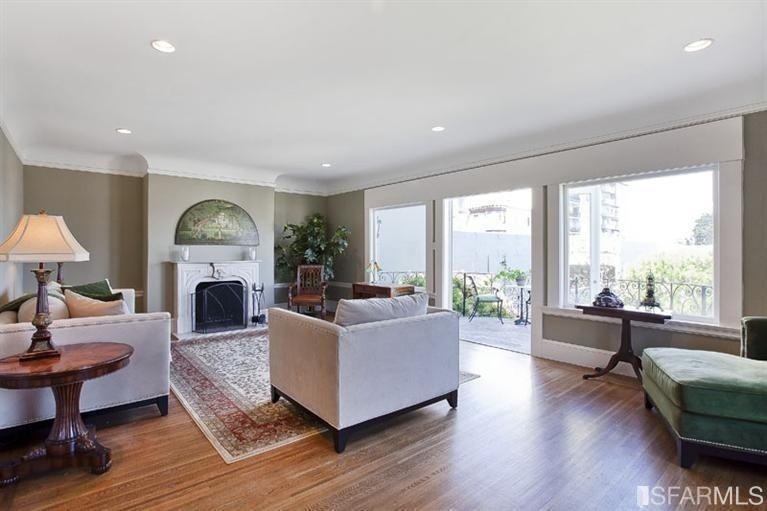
[1,341,133,488]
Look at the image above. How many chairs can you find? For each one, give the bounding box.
[287,264,327,317]
[523,290,531,325]
[468,274,506,325]
[267,305,462,454]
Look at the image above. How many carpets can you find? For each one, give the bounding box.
[169,327,479,466]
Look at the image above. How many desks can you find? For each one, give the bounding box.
[576,305,672,381]
[353,282,414,299]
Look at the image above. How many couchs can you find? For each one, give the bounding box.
[1,285,171,450]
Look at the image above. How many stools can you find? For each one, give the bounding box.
[641,315,767,468]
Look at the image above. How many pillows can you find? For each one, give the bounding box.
[64,286,129,316]
[332,293,429,327]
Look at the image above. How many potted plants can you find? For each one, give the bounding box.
[515,271,528,285]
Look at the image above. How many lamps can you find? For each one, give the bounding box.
[0,210,89,362]
